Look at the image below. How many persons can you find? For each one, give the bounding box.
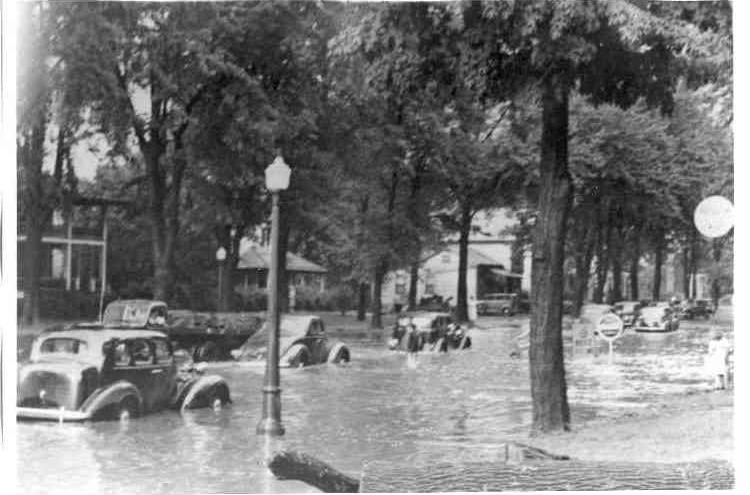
[405,324,421,362]
[707,327,730,390]
[148,310,166,326]
[132,342,155,367]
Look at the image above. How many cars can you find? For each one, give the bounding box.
[580,295,716,336]
[235,314,351,366]
[385,312,455,351]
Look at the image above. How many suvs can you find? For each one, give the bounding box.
[17,329,230,423]
[478,292,518,318]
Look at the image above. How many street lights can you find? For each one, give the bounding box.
[216,247,227,311]
[257,153,294,437]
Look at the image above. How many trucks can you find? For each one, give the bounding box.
[97,300,261,366]
